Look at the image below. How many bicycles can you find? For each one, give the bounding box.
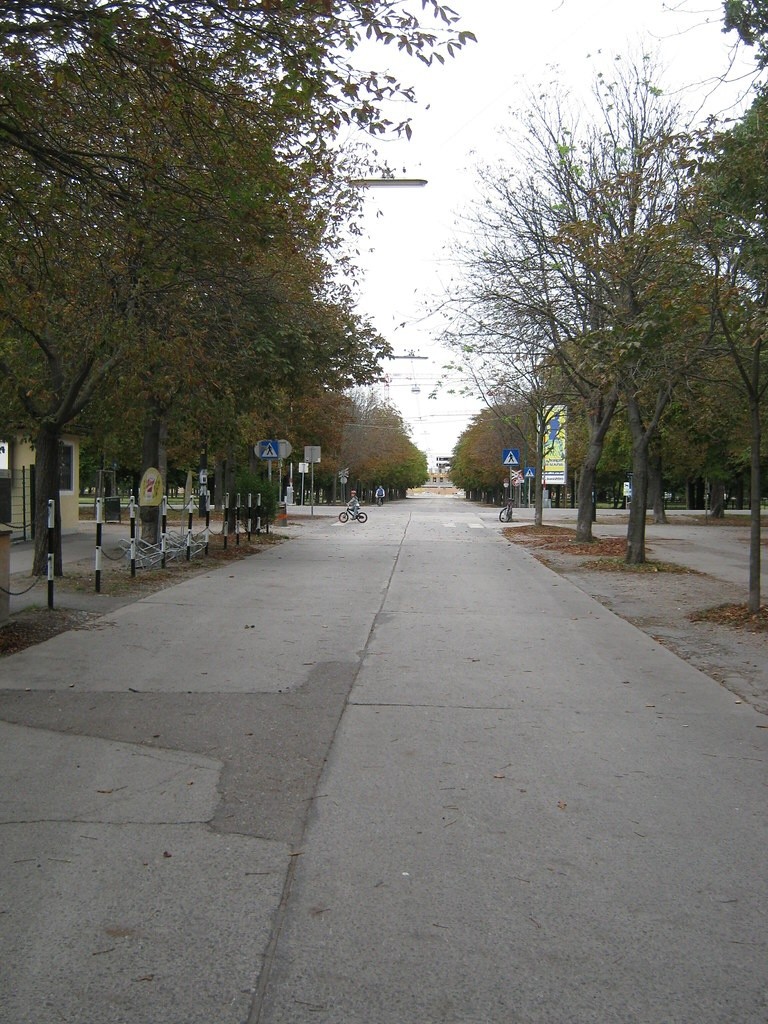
[375,495,384,507]
[498,497,515,522]
[339,502,368,524]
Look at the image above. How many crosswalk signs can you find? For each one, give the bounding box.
[524,467,536,478]
[259,439,278,461]
[502,449,519,466]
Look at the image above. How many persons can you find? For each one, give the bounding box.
[347,490,360,520]
[375,487,385,505]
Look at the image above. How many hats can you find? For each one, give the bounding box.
[351,490,356,494]
